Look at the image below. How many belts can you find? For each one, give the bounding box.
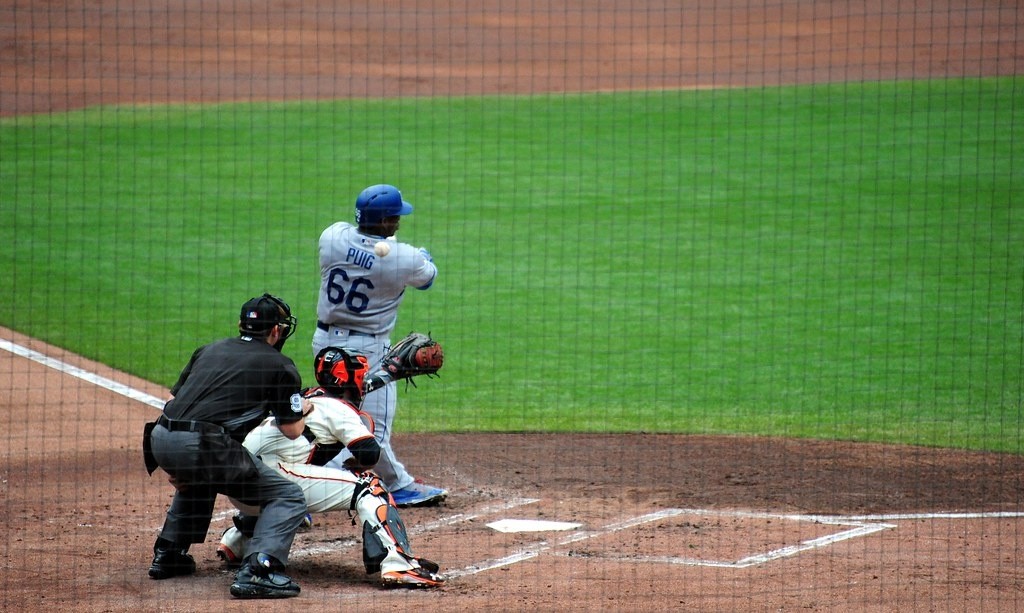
[317,320,376,338]
[158,415,225,435]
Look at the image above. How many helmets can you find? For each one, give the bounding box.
[239,294,292,336]
[315,345,371,404]
[355,185,413,227]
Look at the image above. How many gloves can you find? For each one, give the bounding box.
[418,246,434,262]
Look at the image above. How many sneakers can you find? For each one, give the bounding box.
[381,572,446,590]
[147,548,197,577]
[217,544,240,567]
[298,514,312,528]
[230,553,302,598]
[392,479,449,507]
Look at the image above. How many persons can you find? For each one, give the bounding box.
[312,186,449,507]
[217,334,446,588]
[143,294,315,598]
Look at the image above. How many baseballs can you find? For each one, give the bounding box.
[374,242,390,258]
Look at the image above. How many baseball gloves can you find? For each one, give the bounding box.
[379,330,445,394]
